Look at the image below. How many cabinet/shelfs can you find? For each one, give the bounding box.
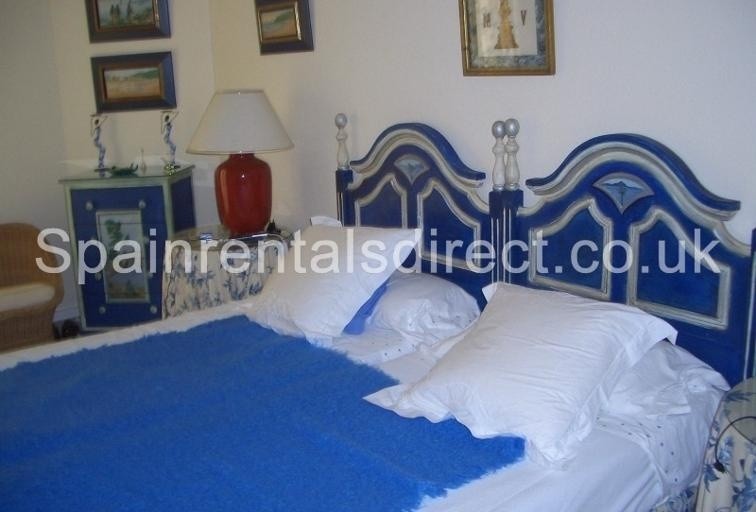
[58,164,195,332]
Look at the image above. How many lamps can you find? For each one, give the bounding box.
[185,87,292,237]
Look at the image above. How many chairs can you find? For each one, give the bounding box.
[0,219,64,355]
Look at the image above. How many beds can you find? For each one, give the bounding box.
[0,115,755,512]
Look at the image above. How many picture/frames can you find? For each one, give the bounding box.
[254,0,314,54]
[91,52,177,113]
[84,0,170,43]
[458,0,556,76]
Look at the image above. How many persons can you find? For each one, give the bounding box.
[493,0,519,49]
[125,0,133,25]
[109,5,120,27]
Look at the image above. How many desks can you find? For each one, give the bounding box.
[694,377,756,512]
[162,225,294,320]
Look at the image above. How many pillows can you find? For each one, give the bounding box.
[366,268,480,348]
[364,280,678,464]
[241,216,421,341]
[420,321,731,419]
